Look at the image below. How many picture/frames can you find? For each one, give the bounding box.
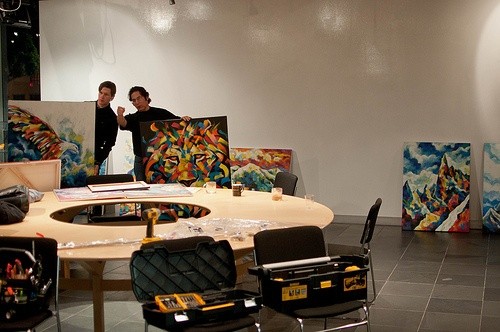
[86,181,150,192]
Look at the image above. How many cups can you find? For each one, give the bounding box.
[272,188,282,201]
[203,182,216,194]
[233,184,244,196]
[305,194,314,210]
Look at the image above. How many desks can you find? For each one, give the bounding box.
[0,184,334,332]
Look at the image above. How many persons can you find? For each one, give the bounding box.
[84,81,119,175]
[117,86,191,181]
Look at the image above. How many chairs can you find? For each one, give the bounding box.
[328,198,383,305]
[0,236,62,332]
[139,235,263,332]
[248,225,373,332]
[270,171,299,195]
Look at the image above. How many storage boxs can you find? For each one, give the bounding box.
[0,248,51,322]
[254,225,367,313]
[130,240,264,332]
